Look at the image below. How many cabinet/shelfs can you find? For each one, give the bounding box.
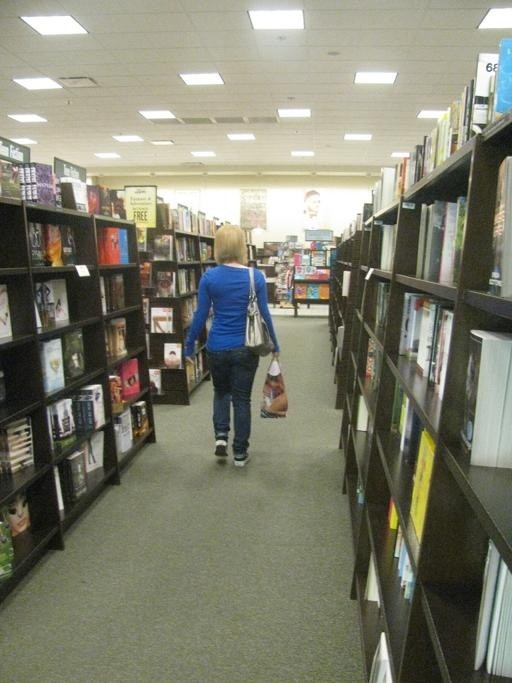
[0,198,157,603]
[292,247,331,316]
[137,221,258,406]
[339,108,512,683]
[328,230,364,409]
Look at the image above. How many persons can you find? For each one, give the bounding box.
[152,315,171,333]
[55,299,65,318]
[53,399,155,525]
[127,375,136,385]
[87,437,96,465]
[4,493,31,535]
[167,352,177,364]
[37,284,51,305]
[303,190,321,229]
[46,357,142,462]
[185,225,280,470]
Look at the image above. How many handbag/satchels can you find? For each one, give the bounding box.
[244,267,276,358]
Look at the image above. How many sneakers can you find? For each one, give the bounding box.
[213,435,228,458]
[234,455,250,470]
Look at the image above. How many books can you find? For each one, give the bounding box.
[39,318,127,393]
[340,52,498,242]
[488,39,512,300]
[415,198,467,286]
[380,223,398,273]
[460,328,511,470]
[373,281,389,326]
[172,203,253,244]
[394,523,414,601]
[0,400,31,576]
[356,476,363,506]
[1,273,126,338]
[258,240,332,309]
[472,539,512,678]
[1,164,126,222]
[399,293,454,400]
[363,551,380,609]
[390,382,437,544]
[25,226,129,267]
[369,632,393,683]
[136,203,182,395]
[357,395,369,432]
